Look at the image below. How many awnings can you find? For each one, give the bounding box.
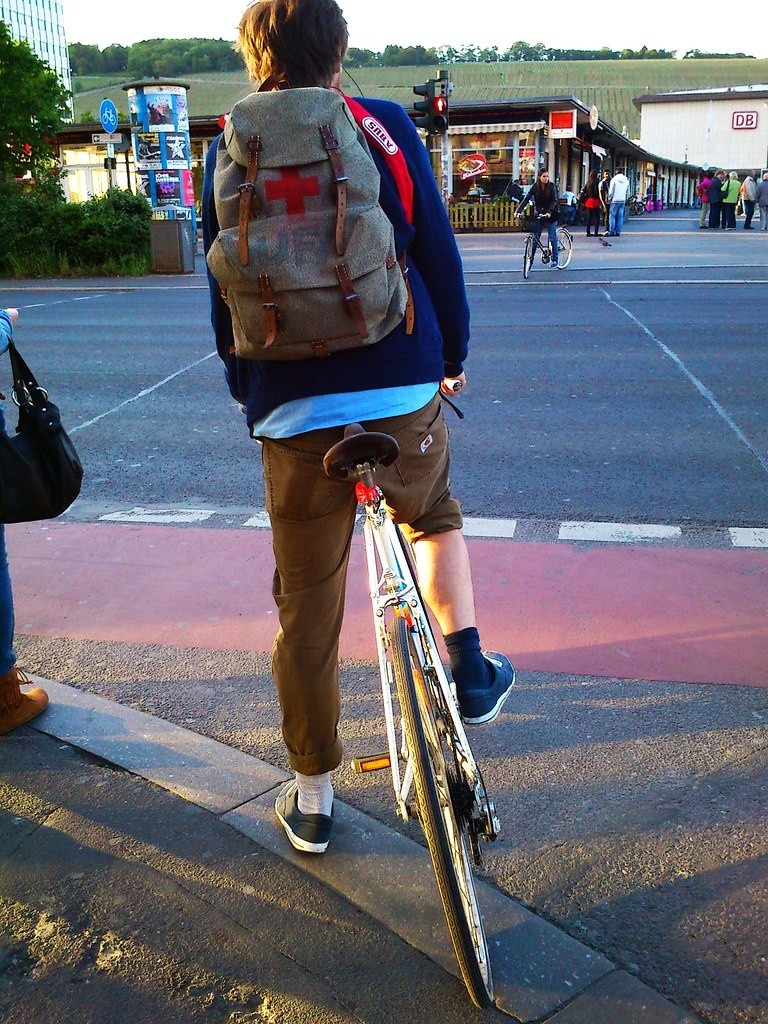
[419,121,544,135]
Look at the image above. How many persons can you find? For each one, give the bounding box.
[507,181,525,204]
[605,169,629,237]
[598,170,612,236]
[514,168,560,268]
[708,169,725,229]
[444,184,484,204]
[0,308,49,736]
[560,184,578,226]
[756,173,768,231]
[148,101,163,125]
[583,169,603,236]
[699,171,714,230]
[740,170,756,230]
[138,137,155,159]
[202,0,516,852]
[721,171,741,231]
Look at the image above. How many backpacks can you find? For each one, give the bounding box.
[206,74,415,362]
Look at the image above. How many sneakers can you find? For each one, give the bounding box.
[551,260,559,269]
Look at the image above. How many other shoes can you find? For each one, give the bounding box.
[699,219,768,231]
[455,650,516,725]
[275,779,335,853]
[587,230,620,236]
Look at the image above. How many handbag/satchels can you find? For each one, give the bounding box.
[1,332,85,524]
[696,186,702,197]
[721,180,730,198]
[577,185,587,203]
[737,199,743,215]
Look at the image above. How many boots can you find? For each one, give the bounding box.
[0,665,50,735]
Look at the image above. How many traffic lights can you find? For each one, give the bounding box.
[432,96,449,131]
[412,79,435,133]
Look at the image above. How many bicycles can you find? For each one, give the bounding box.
[515,213,574,279]
[628,195,646,216]
[321,377,502,1009]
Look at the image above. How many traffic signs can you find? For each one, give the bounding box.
[92,133,123,144]
